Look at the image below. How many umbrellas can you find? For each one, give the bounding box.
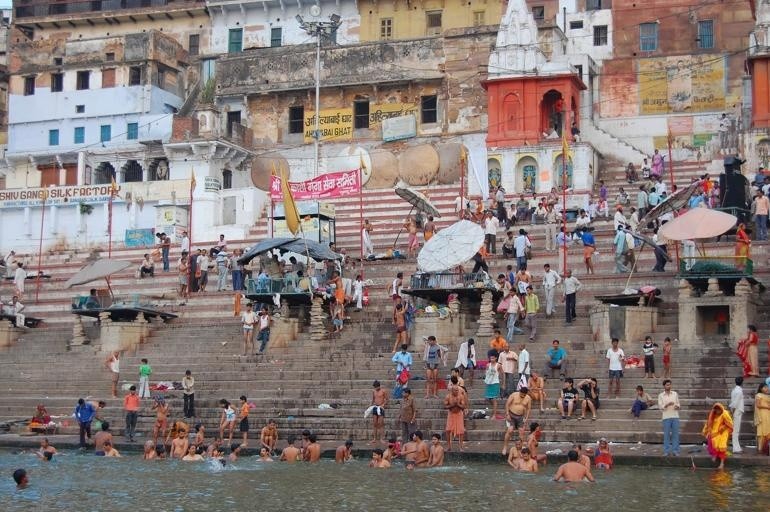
[61,257,133,302]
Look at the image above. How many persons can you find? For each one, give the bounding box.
[5,251,18,277]
[12,468,29,492]
[5,296,26,315]
[85,288,101,308]
[717,112,732,149]
[21,254,33,268]
[672,60,692,101]
[571,121,582,143]
[12,263,27,302]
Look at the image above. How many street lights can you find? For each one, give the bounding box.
[295,10,350,197]
